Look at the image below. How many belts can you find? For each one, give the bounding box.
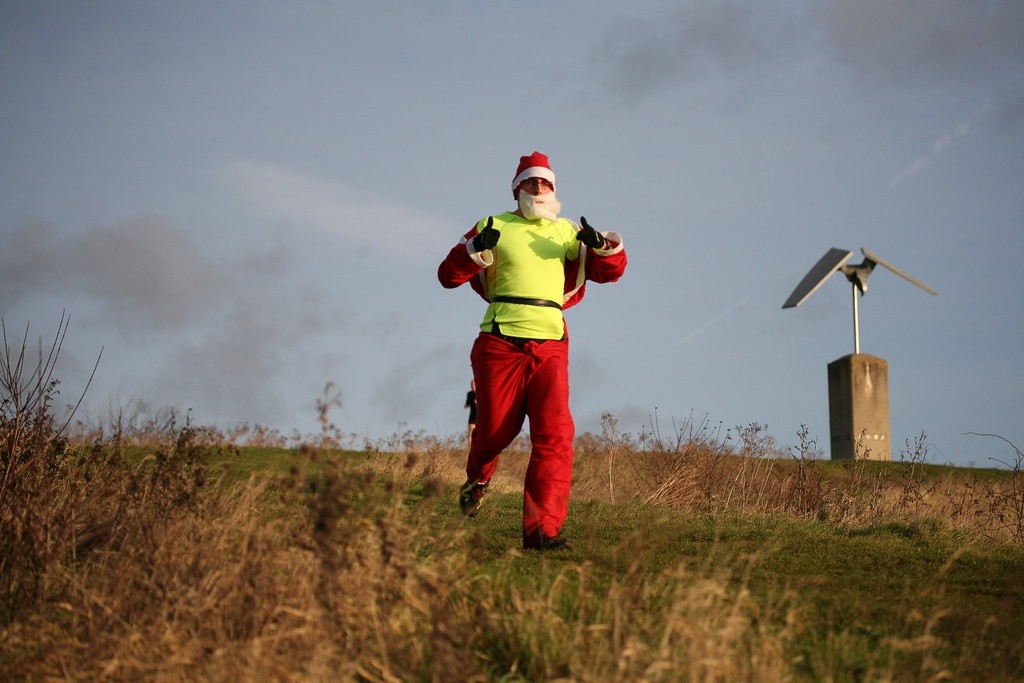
[490,296,562,310]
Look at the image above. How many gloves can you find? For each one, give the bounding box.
[473,216,500,252]
[576,216,604,248]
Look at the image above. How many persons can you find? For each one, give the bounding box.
[436,150,629,554]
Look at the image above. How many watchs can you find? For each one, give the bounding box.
[603,238,611,251]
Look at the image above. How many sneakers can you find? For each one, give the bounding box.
[527,530,573,554]
[460,478,491,518]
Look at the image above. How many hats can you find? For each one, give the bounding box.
[512,151,555,192]
